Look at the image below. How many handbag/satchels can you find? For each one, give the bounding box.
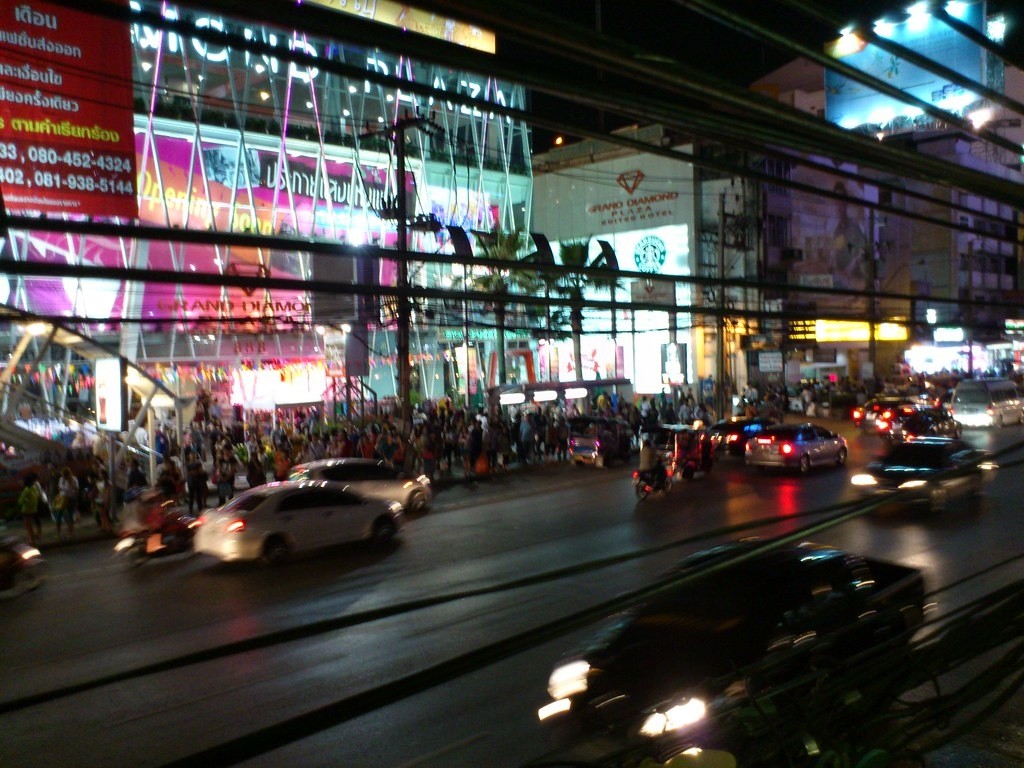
[73,509,80,522]
[220,483,232,496]
[51,493,68,509]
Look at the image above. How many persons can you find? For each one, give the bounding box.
[126,457,147,491]
[830,180,871,278]
[147,388,641,521]
[874,239,897,279]
[85,456,112,536]
[641,358,1024,434]
[18,470,49,546]
[50,466,80,540]
[134,421,150,447]
[0,414,127,460]
[429,198,448,246]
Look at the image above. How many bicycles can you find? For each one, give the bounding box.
[632,459,674,501]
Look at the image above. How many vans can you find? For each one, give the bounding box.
[567,415,633,471]
[952,376,1024,428]
[652,425,718,483]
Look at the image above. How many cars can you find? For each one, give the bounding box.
[851,396,924,431]
[705,415,783,454]
[536,535,933,768]
[291,458,435,514]
[878,404,964,445]
[743,424,851,474]
[863,435,993,508]
[195,479,403,567]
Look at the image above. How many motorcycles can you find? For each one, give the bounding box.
[111,501,202,573]
[1,528,49,599]
